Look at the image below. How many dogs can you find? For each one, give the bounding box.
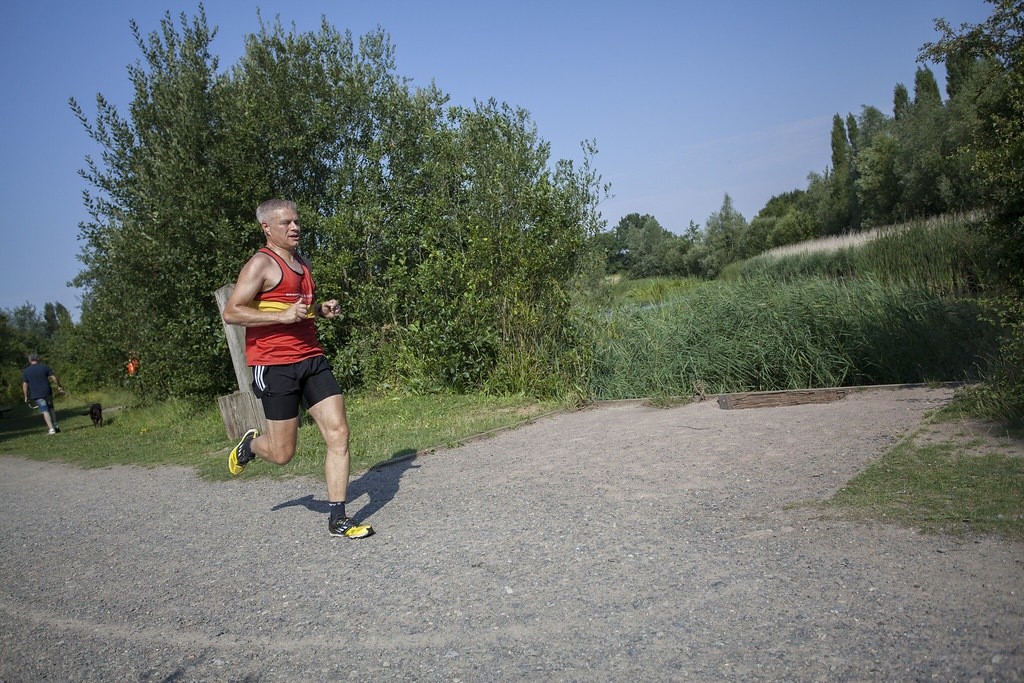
[90,403,103,429]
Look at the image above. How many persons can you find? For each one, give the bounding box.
[22,352,65,437]
[223,200,375,539]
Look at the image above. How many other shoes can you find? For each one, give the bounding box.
[55,428,60,433]
[47,428,55,435]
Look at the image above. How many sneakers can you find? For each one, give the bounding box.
[328,515,374,538]
[229,428,261,475]
